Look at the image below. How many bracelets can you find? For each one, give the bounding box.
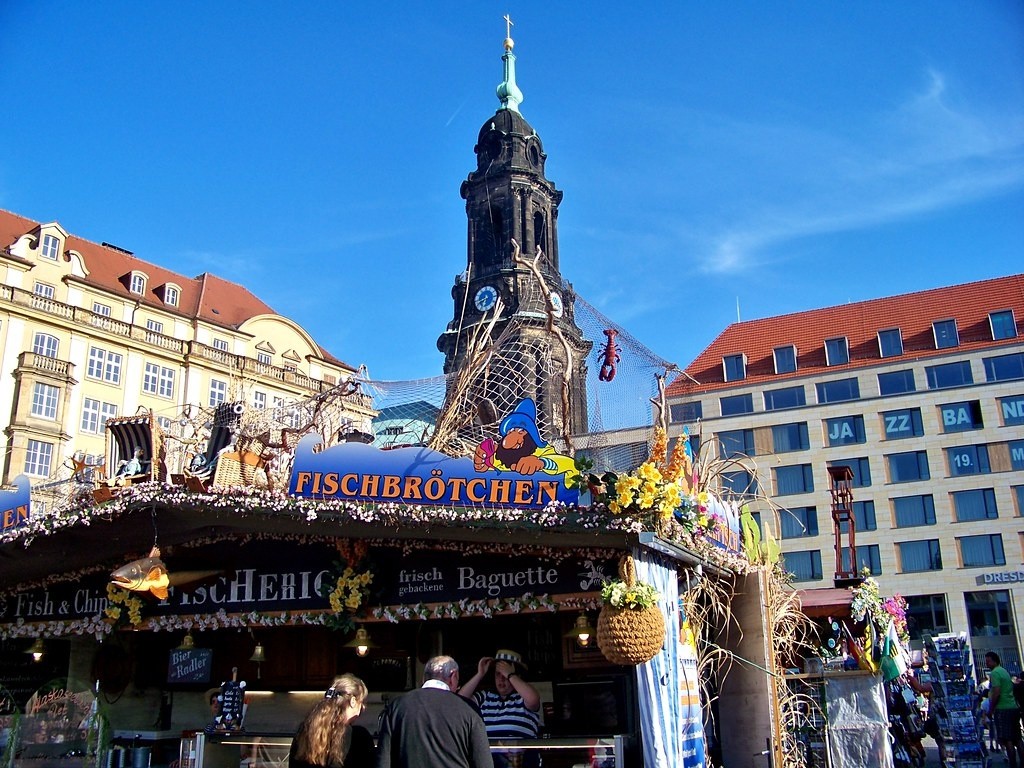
[982,718,985,721]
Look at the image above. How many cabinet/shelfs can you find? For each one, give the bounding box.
[922,630,992,768]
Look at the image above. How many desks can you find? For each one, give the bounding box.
[112,724,197,748]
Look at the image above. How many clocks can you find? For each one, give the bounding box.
[474,286,497,312]
[551,292,564,318]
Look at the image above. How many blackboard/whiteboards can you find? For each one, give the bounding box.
[217,679,246,730]
[166,648,213,684]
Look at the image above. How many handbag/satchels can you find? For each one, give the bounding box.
[901,688,915,704]
[907,702,923,733]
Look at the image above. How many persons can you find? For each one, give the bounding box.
[457,649,540,768]
[168,688,220,768]
[106,446,144,488]
[378,656,494,768]
[886,663,954,768]
[289,672,377,768]
[189,436,238,477]
[972,622,994,636]
[976,652,1024,768]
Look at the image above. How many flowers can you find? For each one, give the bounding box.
[597,579,659,609]
[851,565,911,644]
[0,421,595,632]
[598,455,725,539]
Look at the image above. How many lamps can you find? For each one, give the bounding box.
[249,630,267,665]
[24,637,48,663]
[567,610,594,649]
[174,629,199,651]
[346,624,374,656]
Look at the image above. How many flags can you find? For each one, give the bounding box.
[840,610,907,684]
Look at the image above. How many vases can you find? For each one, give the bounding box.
[594,604,666,666]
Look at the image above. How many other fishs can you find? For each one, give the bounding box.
[109,546,170,600]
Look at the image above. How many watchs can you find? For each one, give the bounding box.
[507,672,516,682]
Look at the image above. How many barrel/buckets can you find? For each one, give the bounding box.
[106,747,129,768]
[128,747,151,768]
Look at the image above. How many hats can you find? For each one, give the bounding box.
[204,687,221,707]
[496,649,528,670]
